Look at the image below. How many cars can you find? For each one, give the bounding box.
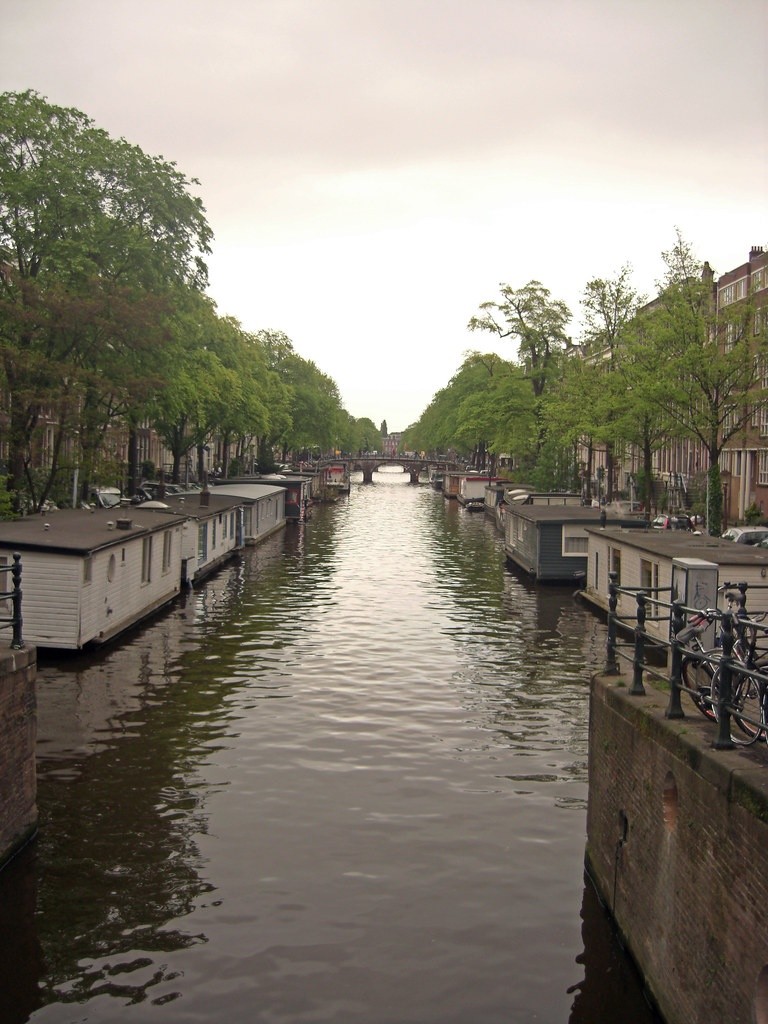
[87,486,125,509]
[717,525,768,545]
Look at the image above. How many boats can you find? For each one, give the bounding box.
[466,501,484,511]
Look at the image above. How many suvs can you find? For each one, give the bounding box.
[652,514,695,533]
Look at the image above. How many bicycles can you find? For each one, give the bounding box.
[676,581,768,746]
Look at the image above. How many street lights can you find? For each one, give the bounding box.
[721,466,730,533]
[614,463,620,501]
